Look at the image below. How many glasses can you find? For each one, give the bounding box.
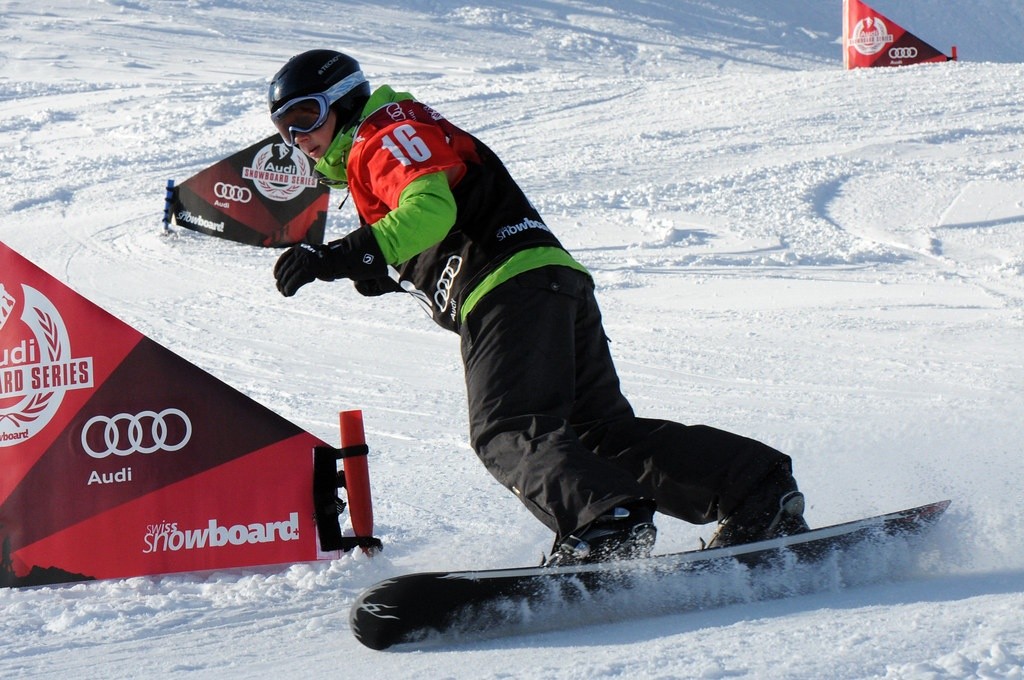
[270,70,368,147]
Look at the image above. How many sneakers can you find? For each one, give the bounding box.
[540,500,657,568]
[702,474,805,551]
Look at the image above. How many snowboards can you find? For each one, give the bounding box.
[348,498,955,653]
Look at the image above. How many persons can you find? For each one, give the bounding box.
[270,50,807,567]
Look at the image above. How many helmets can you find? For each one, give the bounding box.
[267,49,371,120]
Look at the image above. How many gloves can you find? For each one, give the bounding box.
[274,224,392,298]
